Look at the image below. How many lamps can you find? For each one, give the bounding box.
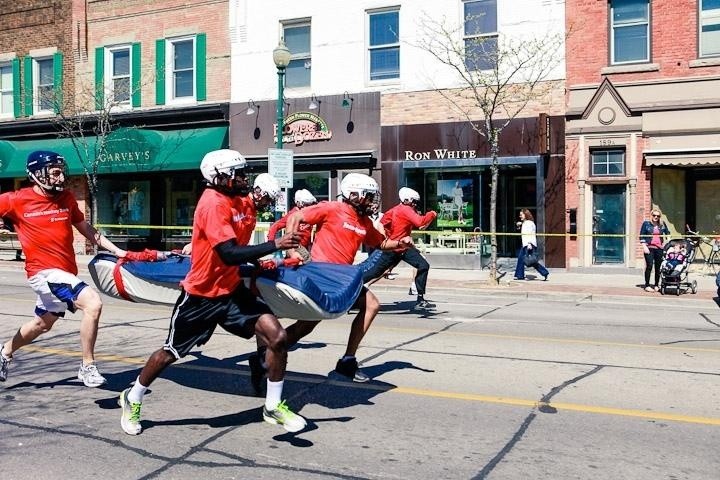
[276,95,290,112]
[342,91,354,108]
[246,98,260,115]
[308,93,322,110]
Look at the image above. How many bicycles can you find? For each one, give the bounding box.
[682,222,720,276]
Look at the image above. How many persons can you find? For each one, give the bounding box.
[248,170,419,393]
[661,243,686,274]
[639,208,668,293]
[265,185,439,312]
[0,150,128,389]
[451,181,465,228]
[120,147,313,439]
[513,207,549,281]
[179,170,281,359]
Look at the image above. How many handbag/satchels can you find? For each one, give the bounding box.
[523,248,539,267]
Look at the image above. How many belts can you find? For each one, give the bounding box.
[648,245,658,249]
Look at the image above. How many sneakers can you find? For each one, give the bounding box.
[78,362,107,387]
[336,358,369,382]
[645,286,661,292]
[0,345,13,382]
[415,301,437,310]
[262,401,307,432]
[120,388,142,435]
[249,355,264,388]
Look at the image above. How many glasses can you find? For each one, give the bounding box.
[652,215,660,217]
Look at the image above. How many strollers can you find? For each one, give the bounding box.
[655,235,699,296]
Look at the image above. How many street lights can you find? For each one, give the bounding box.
[270,35,292,259]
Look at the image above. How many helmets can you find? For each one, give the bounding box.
[294,189,316,208]
[399,186,420,207]
[27,151,71,191]
[253,173,281,210]
[341,173,381,216]
[200,149,250,195]
